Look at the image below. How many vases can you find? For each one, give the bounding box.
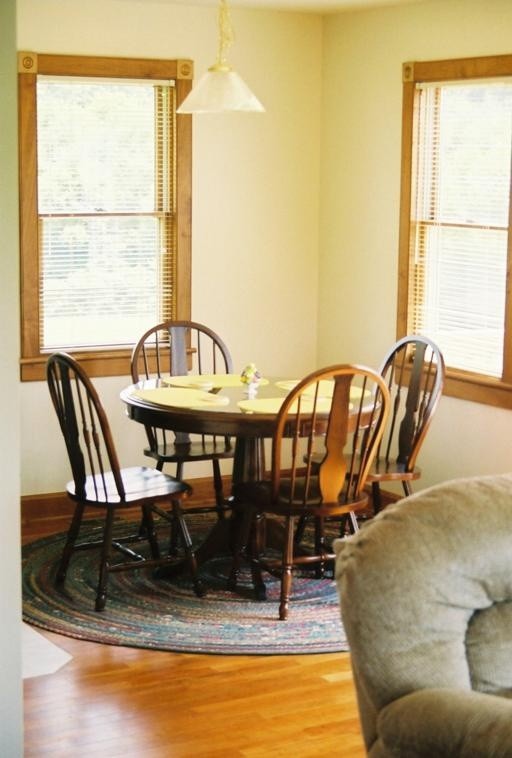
[247,383,259,401]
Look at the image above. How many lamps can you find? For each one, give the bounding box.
[177,0,271,116]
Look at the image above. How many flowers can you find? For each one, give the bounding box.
[240,363,261,385]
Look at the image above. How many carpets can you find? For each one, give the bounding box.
[18,510,364,647]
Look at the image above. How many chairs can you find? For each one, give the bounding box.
[331,475,512,758]
[304,335,444,539]
[130,320,236,556]
[222,363,391,620]
[46,352,208,612]
